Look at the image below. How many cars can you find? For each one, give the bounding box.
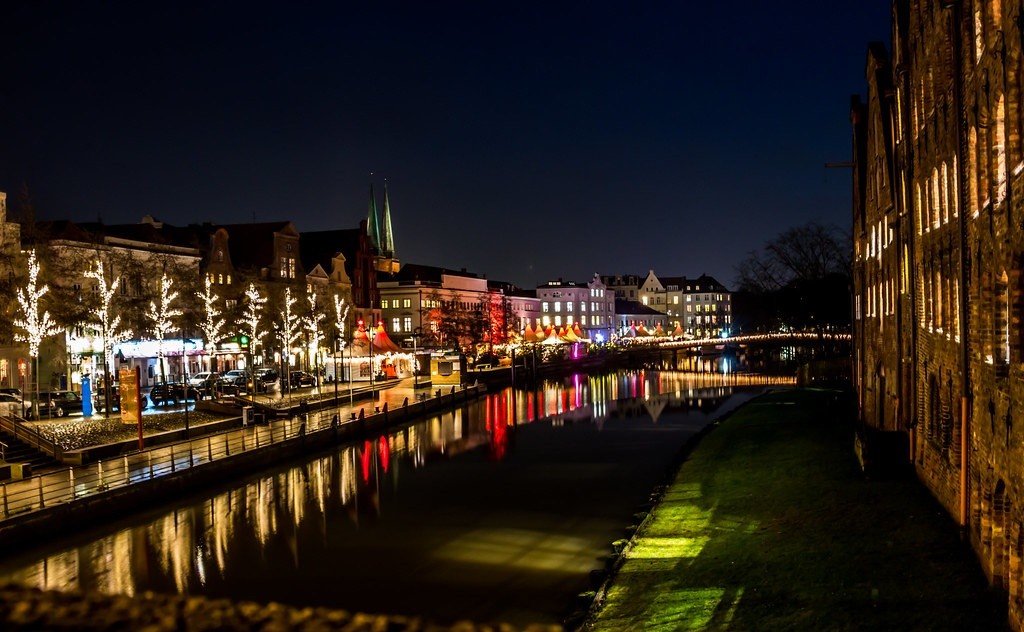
[0,392,33,422]
[217,369,254,397]
[186,372,221,395]
[252,367,278,392]
[288,370,315,389]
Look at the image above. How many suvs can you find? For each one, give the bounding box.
[150,381,203,406]
[36,391,93,418]
[93,384,149,412]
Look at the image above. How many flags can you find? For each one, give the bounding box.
[381,184,394,252]
[367,182,383,256]
